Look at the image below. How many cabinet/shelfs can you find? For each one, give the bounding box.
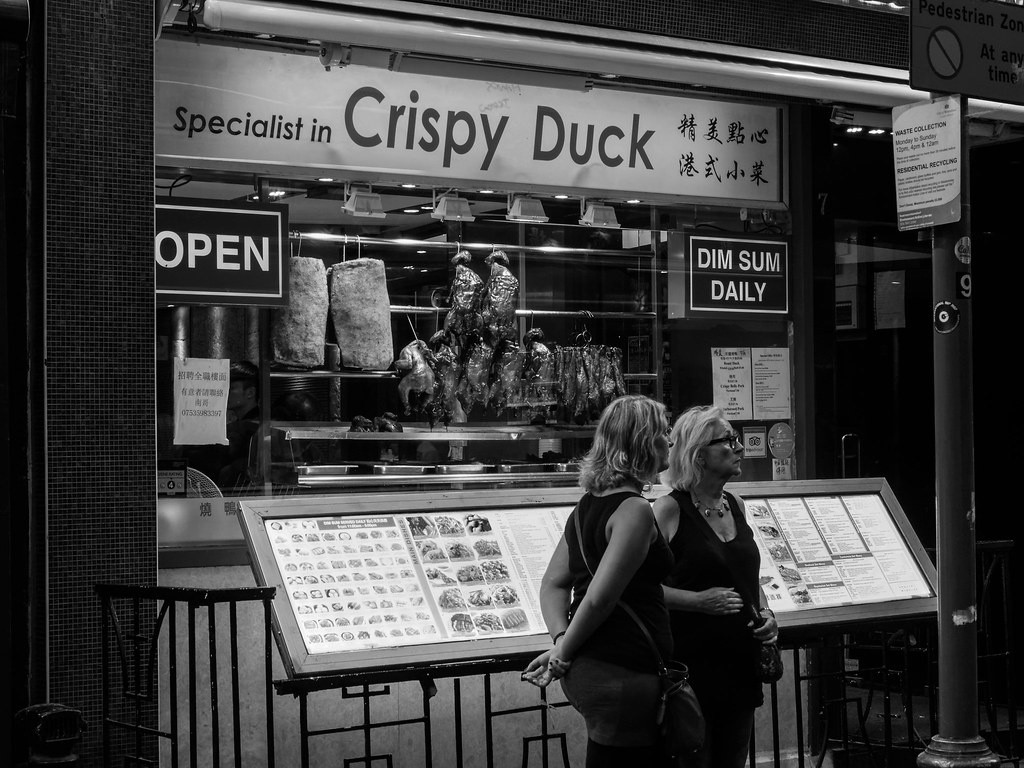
[249,371,602,490]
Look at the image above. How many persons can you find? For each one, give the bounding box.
[226,360,261,442]
[523,395,675,768]
[653,406,778,768]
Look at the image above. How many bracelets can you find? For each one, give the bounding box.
[760,608,775,619]
[553,631,566,645]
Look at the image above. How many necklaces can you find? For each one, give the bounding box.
[692,490,730,518]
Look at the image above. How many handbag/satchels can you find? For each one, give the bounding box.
[749,622,784,684]
[655,659,706,763]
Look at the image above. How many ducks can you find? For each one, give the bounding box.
[395,251,626,425]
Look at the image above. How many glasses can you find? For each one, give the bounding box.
[707,433,740,449]
[660,425,672,438]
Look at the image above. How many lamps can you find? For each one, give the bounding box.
[578,203,621,229]
[340,187,387,219]
[430,197,477,222]
[505,197,549,223]
